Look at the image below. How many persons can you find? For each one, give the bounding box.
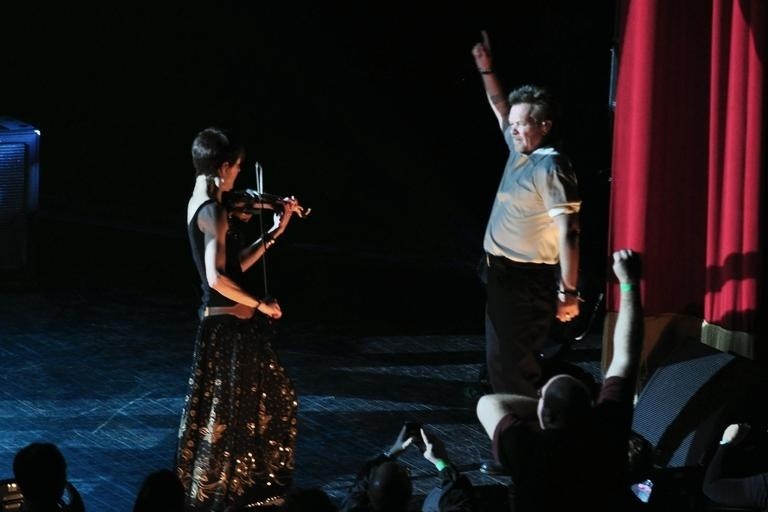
[473,31,584,400]
[175,126,301,509]
[12,441,85,511]
[344,421,481,510]
[476,249,642,508]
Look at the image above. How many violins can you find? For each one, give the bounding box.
[220,187,311,217]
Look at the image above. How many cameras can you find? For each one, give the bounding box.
[401,420,422,449]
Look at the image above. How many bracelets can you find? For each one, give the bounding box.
[260,231,276,250]
[622,281,640,293]
[435,456,453,472]
[478,66,495,75]
[556,284,586,303]
[254,298,261,309]
[382,449,396,459]
